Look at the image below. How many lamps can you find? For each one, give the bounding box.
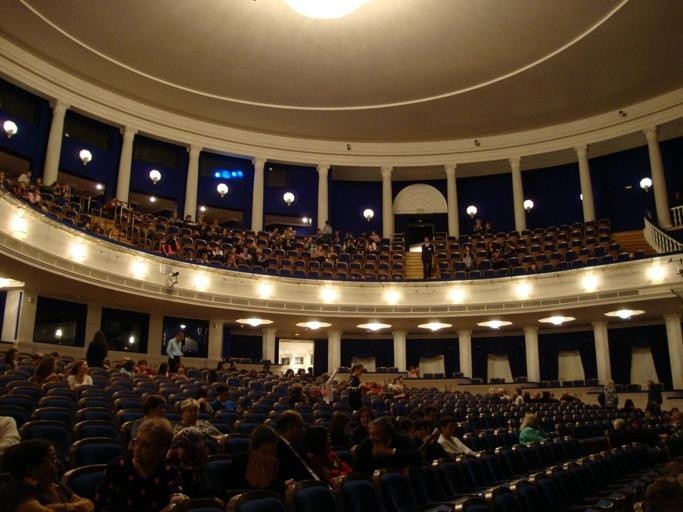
[641,176,655,193]
[466,205,478,220]
[301,216,313,223]
[283,191,296,206]
[149,170,162,184]
[217,183,229,198]
[363,208,374,222]
[524,199,534,212]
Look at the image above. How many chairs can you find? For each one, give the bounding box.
[434,220,646,279]
[36,180,203,265]
[0,350,683,512]
[198,225,407,279]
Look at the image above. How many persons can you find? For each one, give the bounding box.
[0,168,74,205]
[421,237,435,279]
[159,210,380,269]
[103,198,123,213]
[462,218,517,271]
